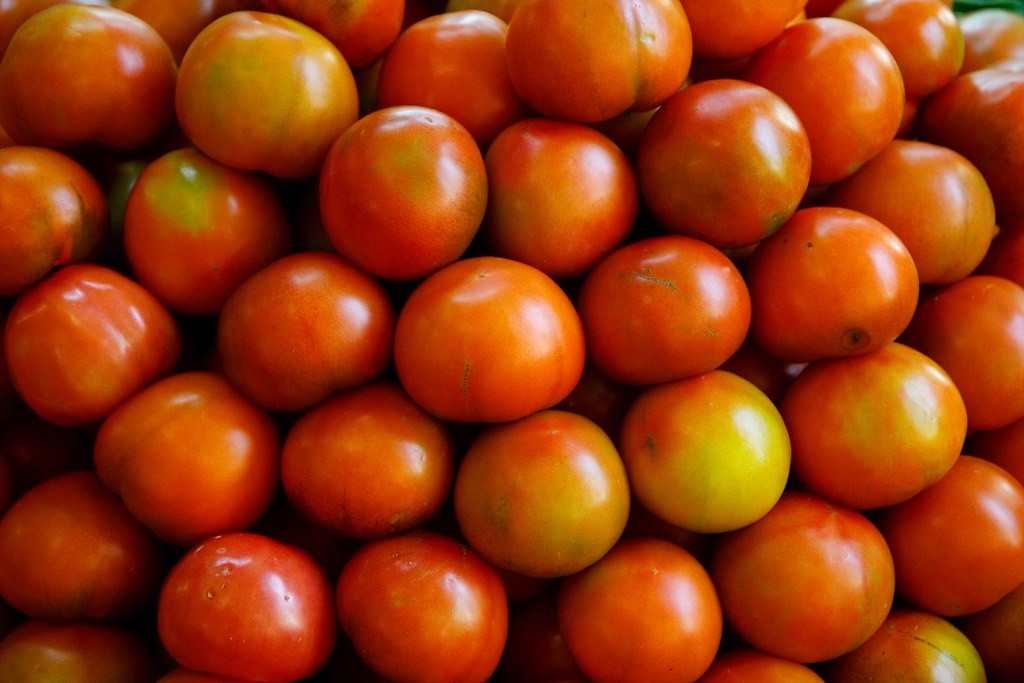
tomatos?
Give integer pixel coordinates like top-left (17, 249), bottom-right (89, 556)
top-left (0, 0), bottom-right (1024, 683)
